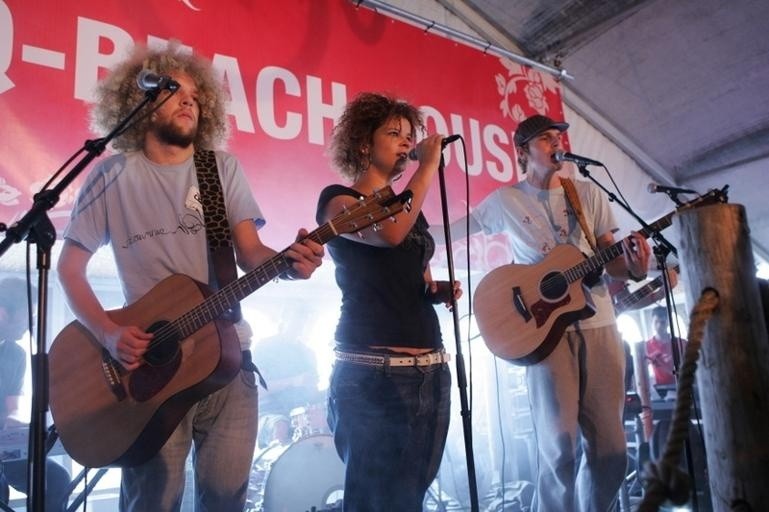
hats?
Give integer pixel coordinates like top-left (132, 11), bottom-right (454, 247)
top-left (515, 114), bottom-right (570, 148)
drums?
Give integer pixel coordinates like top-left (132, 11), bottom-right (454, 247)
top-left (290, 401), bottom-right (329, 439)
top-left (245, 433), bottom-right (347, 512)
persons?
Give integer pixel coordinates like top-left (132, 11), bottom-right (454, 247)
top-left (572, 263), bottom-right (682, 481)
top-left (312, 89), bottom-right (464, 512)
top-left (55, 38), bottom-right (326, 512)
top-left (424, 112), bottom-right (654, 511)
top-left (1, 272), bottom-right (73, 512)
top-left (246, 313), bottom-right (324, 457)
top-left (628, 302), bottom-right (689, 449)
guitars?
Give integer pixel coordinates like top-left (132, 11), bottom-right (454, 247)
top-left (48, 186), bottom-right (411, 466)
top-left (473, 185), bottom-right (730, 364)
top-left (610, 264), bottom-right (680, 320)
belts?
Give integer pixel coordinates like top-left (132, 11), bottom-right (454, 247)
top-left (334, 347), bottom-right (451, 368)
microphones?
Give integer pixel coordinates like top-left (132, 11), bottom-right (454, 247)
top-left (552, 145), bottom-right (600, 170)
top-left (644, 180), bottom-right (689, 199)
top-left (407, 134), bottom-right (463, 162)
top-left (133, 66), bottom-right (173, 92)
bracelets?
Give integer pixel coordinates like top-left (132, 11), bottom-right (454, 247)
top-left (625, 269), bottom-right (648, 284)
top-left (278, 272), bottom-right (293, 282)
